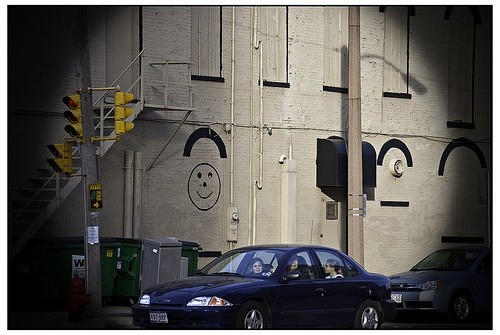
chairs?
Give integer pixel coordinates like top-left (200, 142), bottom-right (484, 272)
top-left (297, 256), bottom-right (313, 279)
top-left (263, 264), bottom-right (273, 273)
top-left (322, 264), bottom-right (348, 278)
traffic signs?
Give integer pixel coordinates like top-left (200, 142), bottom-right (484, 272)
top-left (89, 182), bottom-right (104, 211)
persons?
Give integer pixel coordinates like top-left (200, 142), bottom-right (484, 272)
top-left (324, 259), bottom-right (345, 279)
top-left (246, 258), bottom-right (270, 277)
top-left (286, 252), bottom-right (298, 272)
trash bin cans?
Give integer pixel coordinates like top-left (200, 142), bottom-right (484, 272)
top-left (114, 236), bottom-right (143, 307)
top-left (179, 240), bottom-right (203, 274)
top-left (26, 235), bottom-right (115, 311)
top-left (141, 235), bottom-right (182, 299)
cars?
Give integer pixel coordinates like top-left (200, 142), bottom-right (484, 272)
top-left (387, 244), bottom-right (493, 330)
top-left (131, 219), bottom-right (398, 330)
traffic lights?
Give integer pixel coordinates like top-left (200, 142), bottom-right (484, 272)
top-left (47, 144), bottom-right (72, 173)
top-left (62, 95), bottom-right (84, 139)
top-left (114, 91), bottom-right (135, 136)
